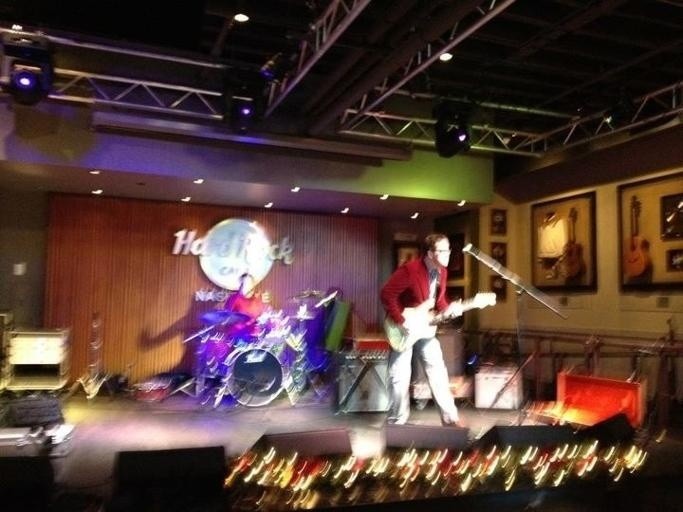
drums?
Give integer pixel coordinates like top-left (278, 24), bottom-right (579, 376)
top-left (224, 342), bottom-right (289, 406)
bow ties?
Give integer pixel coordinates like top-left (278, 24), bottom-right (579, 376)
top-left (429, 269), bottom-right (439, 282)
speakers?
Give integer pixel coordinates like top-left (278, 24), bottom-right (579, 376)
top-left (476, 424), bottom-right (575, 451)
top-left (385, 423), bottom-right (470, 451)
top-left (0, 398), bottom-right (63, 427)
top-left (115, 445), bottom-right (225, 512)
top-left (240, 429), bottom-right (354, 461)
top-left (575, 413), bottom-right (635, 448)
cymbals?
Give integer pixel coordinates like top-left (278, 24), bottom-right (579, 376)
top-left (203, 311), bottom-right (251, 323)
top-left (290, 291), bottom-right (321, 300)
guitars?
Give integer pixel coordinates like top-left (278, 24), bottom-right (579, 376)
top-left (621, 196), bottom-right (651, 278)
top-left (384, 293), bottom-right (496, 352)
top-left (559, 208), bottom-right (587, 283)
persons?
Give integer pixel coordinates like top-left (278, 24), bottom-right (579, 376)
top-left (378, 233), bottom-right (464, 428)
top-left (224, 273), bottom-right (271, 346)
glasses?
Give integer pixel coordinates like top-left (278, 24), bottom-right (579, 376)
top-left (433, 249), bottom-right (451, 255)
